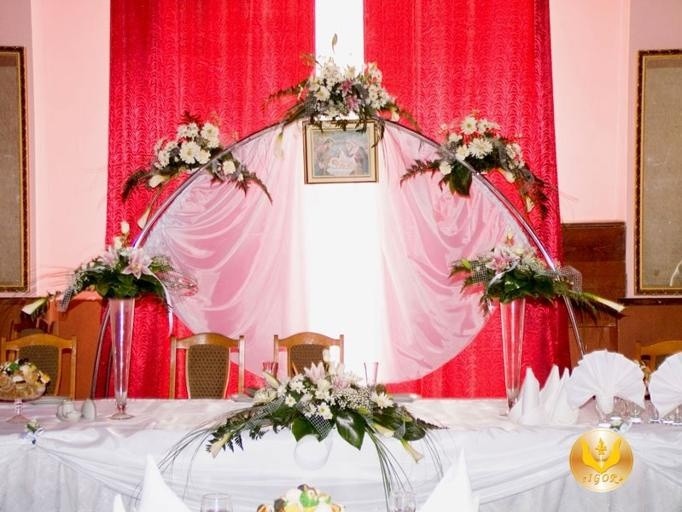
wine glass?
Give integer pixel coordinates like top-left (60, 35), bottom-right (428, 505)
top-left (0, 382), bottom-right (46, 425)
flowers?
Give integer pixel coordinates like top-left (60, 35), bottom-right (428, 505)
top-left (451, 232), bottom-right (626, 321)
top-left (400, 109), bottom-right (548, 222)
top-left (56, 220), bottom-right (197, 313)
top-left (134, 346), bottom-right (443, 511)
top-left (121, 112), bottom-right (272, 231)
top-left (259, 32), bottom-right (420, 150)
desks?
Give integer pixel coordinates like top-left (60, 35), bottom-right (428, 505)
top-left (0, 395), bottom-right (681, 512)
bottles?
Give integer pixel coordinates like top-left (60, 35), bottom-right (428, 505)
top-left (80, 397), bottom-right (98, 423)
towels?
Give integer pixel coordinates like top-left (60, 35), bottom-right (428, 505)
top-left (511, 364), bottom-right (579, 425)
top-left (565, 349), bottom-right (646, 415)
top-left (647, 352), bottom-right (681, 418)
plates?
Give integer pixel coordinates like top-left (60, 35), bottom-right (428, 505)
top-left (388, 393), bottom-right (422, 403)
top-left (229, 393), bottom-right (253, 403)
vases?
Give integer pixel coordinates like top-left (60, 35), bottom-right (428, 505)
top-left (499, 296), bottom-right (526, 416)
top-left (105, 298), bottom-right (139, 420)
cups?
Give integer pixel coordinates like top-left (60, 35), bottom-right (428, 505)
top-left (596, 396), bottom-right (682, 431)
top-left (67, 411), bottom-right (82, 422)
top-left (364, 360), bottom-right (379, 391)
top-left (262, 362), bottom-right (278, 382)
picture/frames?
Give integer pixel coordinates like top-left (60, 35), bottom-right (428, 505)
top-left (0, 45), bottom-right (31, 292)
top-left (302, 119), bottom-right (379, 184)
top-left (633, 47), bottom-right (682, 296)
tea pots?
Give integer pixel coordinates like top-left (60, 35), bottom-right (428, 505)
top-left (57, 398), bottom-right (75, 418)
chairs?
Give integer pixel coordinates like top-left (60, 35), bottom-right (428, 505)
top-left (634, 340), bottom-right (682, 394)
top-left (275, 332), bottom-right (344, 395)
top-left (1, 334), bottom-right (78, 402)
top-left (168, 332), bottom-right (245, 400)
top-left (8, 319), bottom-right (53, 363)
